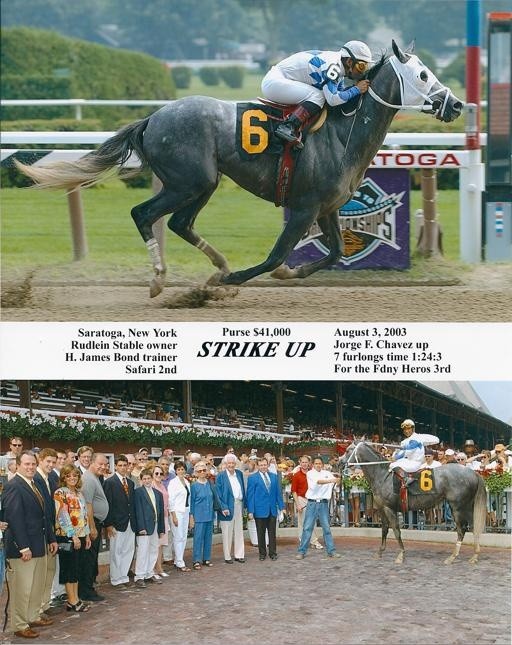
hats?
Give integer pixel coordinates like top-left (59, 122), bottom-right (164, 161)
top-left (444, 449), bottom-right (455, 456)
top-left (495, 444), bottom-right (505, 451)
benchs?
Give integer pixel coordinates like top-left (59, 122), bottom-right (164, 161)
top-left (1, 380), bottom-right (325, 437)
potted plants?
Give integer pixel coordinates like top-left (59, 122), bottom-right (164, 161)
top-left (484, 470), bottom-right (511, 496)
top-left (280, 476), bottom-right (291, 493)
top-left (341, 475), bottom-right (373, 496)
top-left (242, 509), bottom-right (269, 549)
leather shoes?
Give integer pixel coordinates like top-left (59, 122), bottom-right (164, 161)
top-left (14, 628), bottom-right (38, 637)
top-left (30, 615), bottom-right (53, 626)
top-left (88, 594), bottom-right (104, 601)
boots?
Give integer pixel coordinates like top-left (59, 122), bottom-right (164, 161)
top-left (395, 467), bottom-right (416, 487)
top-left (275, 101), bottom-right (322, 149)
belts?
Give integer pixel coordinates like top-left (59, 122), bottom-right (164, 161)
top-left (308, 499), bottom-right (328, 503)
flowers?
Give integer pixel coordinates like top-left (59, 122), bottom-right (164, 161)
top-left (1, 409), bottom-right (334, 458)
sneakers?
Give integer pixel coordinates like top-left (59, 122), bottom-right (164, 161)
top-left (135, 578), bottom-right (147, 588)
top-left (154, 572), bottom-right (170, 579)
top-left (329, 552), bottom-right (343, 558)
top-left (295, 553), bottom-right (304, 559)
top-left (270, 554), bottom-right (277, 560)
top-left (113, 582), bottom-right (136, 591)
top-left (259, 555), bottom-right (266, 561)
top-left (235, 557), bottom-right (245, 563)
top-left (145, 576), bottom-right (163, 584)
top-left (224, 560), bottom-right (234, 564)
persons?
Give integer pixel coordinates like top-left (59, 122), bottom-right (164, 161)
top-left (262, 40), bottom-right (374, 146)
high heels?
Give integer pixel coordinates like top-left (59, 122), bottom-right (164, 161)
top-left (174, 564), bottom-right (192, 572)
top-left (67, 599), bottom-right (88, 612)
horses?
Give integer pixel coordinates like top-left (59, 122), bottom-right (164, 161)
top-left (335, 433), bottom-right (487, 565)
top-left (12, 40), bottom-right (463, 299)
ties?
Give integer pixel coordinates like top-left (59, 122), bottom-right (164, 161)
top-left (122, 478), bottom-right (129, 495)
top-left (31, 481), bottom-right (45, 512)
top-left (263, 472), bottom-right (270, 489)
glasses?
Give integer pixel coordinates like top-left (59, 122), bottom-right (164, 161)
top-left (11, 443), bottom-right (22, 447)
top-left (154, 472), bottom-right (164, 476)
top-left (425, 455), bottom-right (432, 458)
top-left (481, 456), bottom-right (487, 459)
top-left (196, 469), bottom-right (206, 473)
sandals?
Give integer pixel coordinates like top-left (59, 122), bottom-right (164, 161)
top-left (203, 560), bottom-right (213, 567)
top-left (193, 562), bottom-right (202, 570)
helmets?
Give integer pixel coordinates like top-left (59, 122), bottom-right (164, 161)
top-left (341, 40), bottom-right (375, 63)
top-left (400, 419), bottom-right (416, 428)
